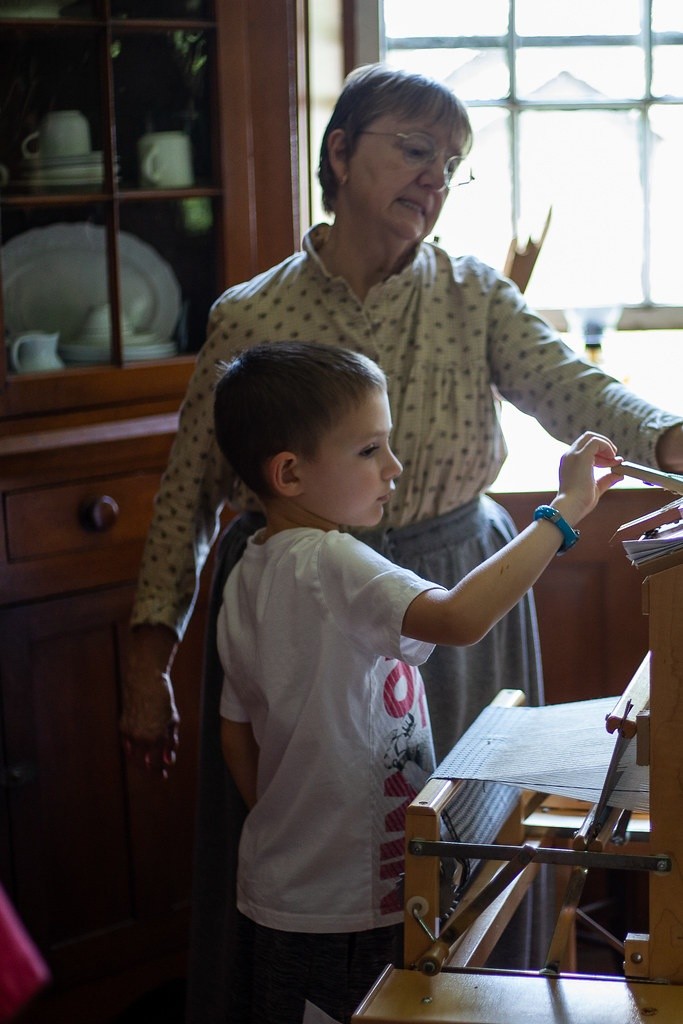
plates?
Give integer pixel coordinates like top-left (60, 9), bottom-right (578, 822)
top-left (0, 152), bottom-right (184, 363)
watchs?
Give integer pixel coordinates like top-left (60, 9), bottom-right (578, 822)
top-left (533, 505), bottom-right (581, 556)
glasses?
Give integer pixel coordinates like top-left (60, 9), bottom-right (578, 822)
top-left (354, 131), bottom-right (477, 187)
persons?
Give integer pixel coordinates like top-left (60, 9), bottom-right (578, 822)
top-left (214, 340), bottom-right (624, 1024)
top-left (118, 70), bottom-right (682, 1024)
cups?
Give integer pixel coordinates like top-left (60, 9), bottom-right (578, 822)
top-left (5, 328), bottom-right (66, 377)
top-left (137, 130), bottom-right (194, 190)
top-left (22, 112), bottom-right (91, 160)
